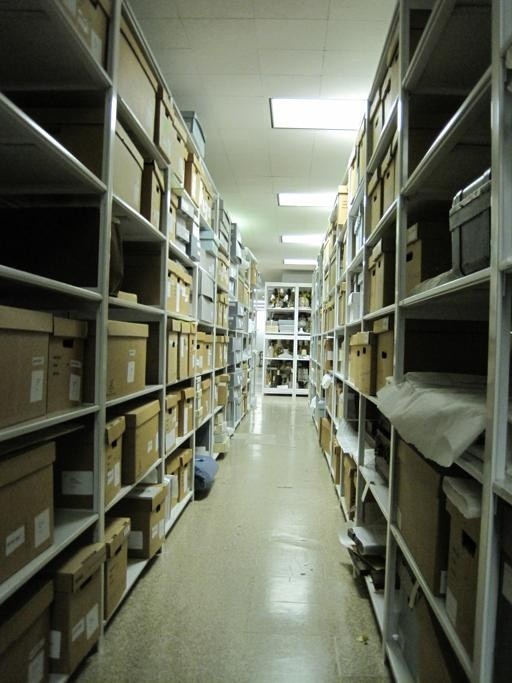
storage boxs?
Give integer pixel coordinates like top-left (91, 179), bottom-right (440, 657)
top-left (0, 0), bottom-right (258, 680)
top-left (305, 0), bottom-right (511, 679)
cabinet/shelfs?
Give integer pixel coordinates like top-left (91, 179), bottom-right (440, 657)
top-left (262, 281), bottom-right (316, 396)
